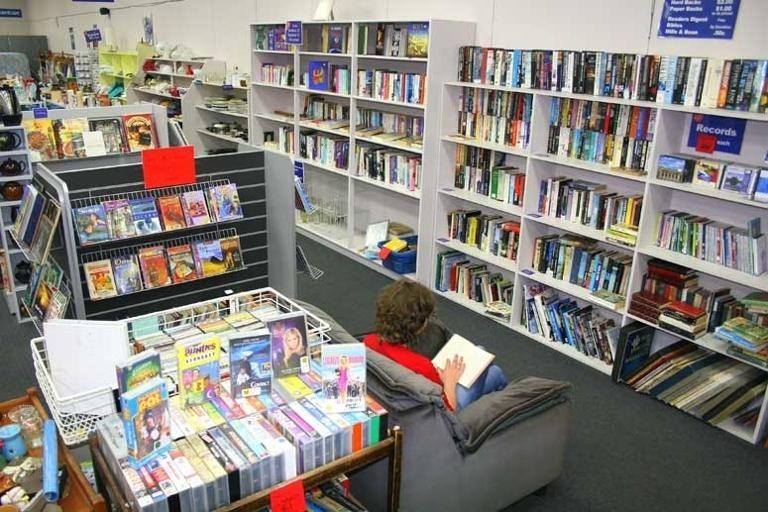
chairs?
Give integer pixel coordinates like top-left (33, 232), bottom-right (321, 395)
top-left (287, 294), bottom-right (573, 510)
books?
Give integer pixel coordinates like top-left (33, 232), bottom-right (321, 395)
top-left (255, 22), bottom-right (430, 191)
top-left (611, 320), bottom-right (768, 425)
top-left (26, 255), bottom-right (389, 512)
top-left (23, 113), bottom-right (243, 301)
top-left (435, 249), bottom-right (515, 323)
top-left (430, 333), bottom-right (496, 389)
top-left (364, 219), bottom-right (417, 261)
top-left (628, 258), bottom-right (768, 368)
top-left (458, 46), bottom-right (768, 312)
top-left (520, 282), bottom-right (621, 365)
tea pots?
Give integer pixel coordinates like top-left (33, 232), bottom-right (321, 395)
top-left (0, 131), bottom-right (32, 284)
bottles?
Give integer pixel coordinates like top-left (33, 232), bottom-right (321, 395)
top-left (0, 406), bottom-right (46, 462)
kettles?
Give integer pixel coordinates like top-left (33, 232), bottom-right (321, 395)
top-left (0, 84), bottom-right (22, 125)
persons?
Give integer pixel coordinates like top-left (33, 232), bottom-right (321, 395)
top-left (362, 275), bottom-right (506, 412)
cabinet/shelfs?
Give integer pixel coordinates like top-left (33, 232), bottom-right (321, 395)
top-left (432, 81), bottom-right (768, 444)
top-left (249, 19), bottom-right (477, 285)
top-left (42, 50), bottom-right (89, 88)
top-left (1, 386), bottom-right (109, 512)
top-left (126, 57), bottom-right (225, 145)
top-left (98, 49), bottom-right (153, 103)
top-left (194, 80), bottom-right (248, 150)
